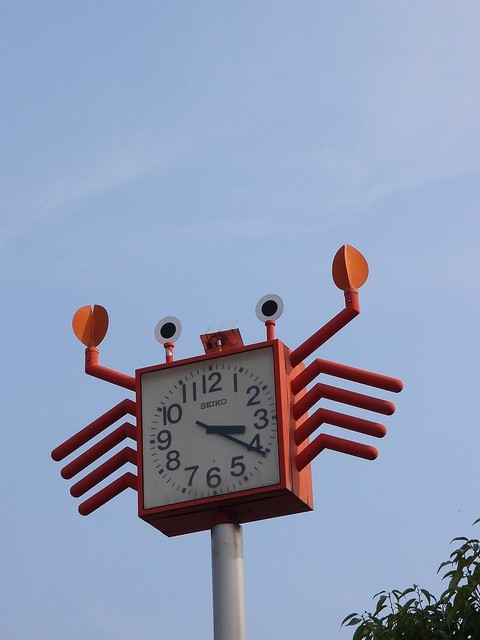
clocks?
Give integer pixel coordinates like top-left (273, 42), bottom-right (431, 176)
top-left (140, 345), bottom-right (281, 512)
